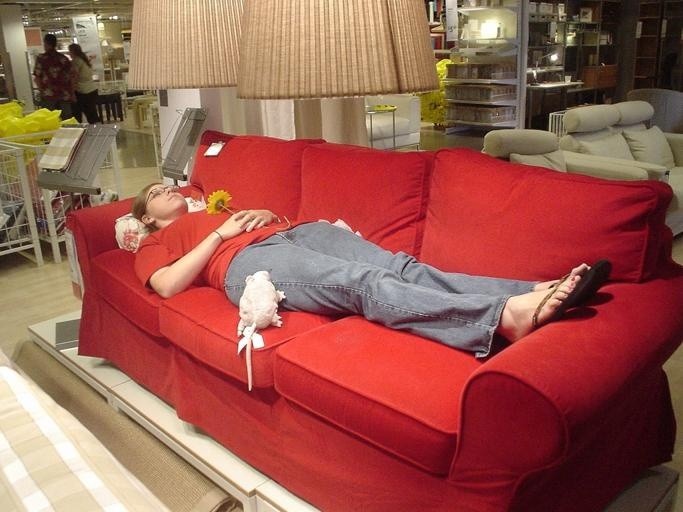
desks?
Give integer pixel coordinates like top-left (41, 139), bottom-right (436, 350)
top-left (96, 88), bottom-right (125, 124)
top-left (527, 81), bottom-right (584, 129)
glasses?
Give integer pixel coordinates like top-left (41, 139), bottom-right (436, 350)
top-left (146, 184), bottom-right (180, 205)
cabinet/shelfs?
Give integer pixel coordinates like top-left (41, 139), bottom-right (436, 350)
top-left (526, 3), bottom-right (565, 78)
top-left (564, 20), bottom-right (601, 107)
top-left (657, 1), bottom-right (683, 91)
top-left (443, 4), bottom-right (522, 130)
top-left (631, 1), bottom-right (663, 88)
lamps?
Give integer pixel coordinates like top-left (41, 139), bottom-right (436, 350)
top-left (234, 1), bottom-right (442, 103)
top-left (530, 50), bottom-right (559, 87)
top-left (126, 1), bottom-right (242, 90)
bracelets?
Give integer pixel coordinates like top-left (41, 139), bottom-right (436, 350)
top-left (213, 231), bottom-right (224, 242)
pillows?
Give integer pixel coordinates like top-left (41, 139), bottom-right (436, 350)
top-left (507, 149), bottom-right (569, 176)
top-left (578, 133), bottom-right (635, 165)
top-left (622, 125), bottom-right (676, 172)
top-left (109, 196), bottom-right (205, 253)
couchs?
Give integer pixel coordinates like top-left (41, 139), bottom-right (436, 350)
top-left (364, 94), bottom-right (422, 153)
top-left (626, 87), bottom-right (683, 133)
top-left (560, 99), bottom-right (683, 238)
top-left (481, 126), bottom-right (649, 188)
top-left (65, 129), bottom-right (683, 509)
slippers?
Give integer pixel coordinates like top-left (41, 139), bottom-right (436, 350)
top-left (528, 269), bottom-right (596, 327)
top-left (549, 259), bottom-right (612, 300)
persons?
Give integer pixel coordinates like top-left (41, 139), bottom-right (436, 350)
top-left (68, 43), bottom-right (100, 123)
top-left (131, 182), bottom-right (612, 360)
top-left (32, 34), bottom-right (72, 121)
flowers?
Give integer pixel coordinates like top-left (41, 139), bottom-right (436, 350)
top-left (204, 188), bottom-right (236, 219)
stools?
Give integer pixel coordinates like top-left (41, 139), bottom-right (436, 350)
top-left (130, 95), bottom-right (155, 129)
top-left (363, 105), bottom-right (397, 152)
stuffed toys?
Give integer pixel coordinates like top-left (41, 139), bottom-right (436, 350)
top-left (237, 270), bottom-right (285, 391)
